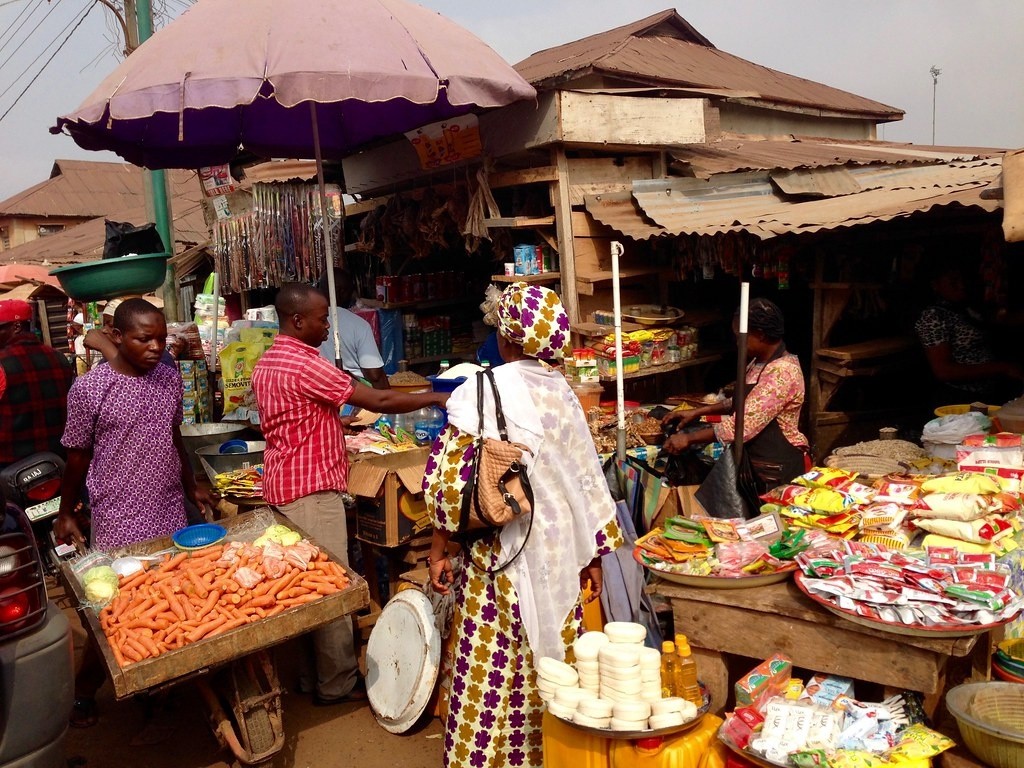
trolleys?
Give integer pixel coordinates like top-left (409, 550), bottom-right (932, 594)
top-left (63, 497), bottom-right (371, 768)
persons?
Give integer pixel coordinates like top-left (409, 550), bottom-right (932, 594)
top-left (914, 257), bottom-right (1024, 417)
top-left (310, 266), bottom-right (391, 392)
top-left (250, 282), bottom-right (451, 702)
top-left (1, 299), bottom-right (77, 531)
top-left (661, 298), bottom-right (816, 497)
top-left (83, 299), bottom-right (177, 370)
top-left (423, 281), bottom-right (624, 768)
top-left (53, 298), bottom-right (217, 731)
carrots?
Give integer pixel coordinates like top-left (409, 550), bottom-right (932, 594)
top-left (98, 542), bottom-right (351, 669)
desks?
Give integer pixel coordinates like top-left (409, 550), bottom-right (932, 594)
top-left (642, 577), bottom-right (985, 728)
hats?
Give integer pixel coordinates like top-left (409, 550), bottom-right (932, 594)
top-left (0, 299), bottom-right (33, 325)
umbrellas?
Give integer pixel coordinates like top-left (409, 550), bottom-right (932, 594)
top-left (52, 0), bottom-right (539, 372)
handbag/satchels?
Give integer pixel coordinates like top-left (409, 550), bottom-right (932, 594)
top-left (466, 370), bottom-right (532, 530)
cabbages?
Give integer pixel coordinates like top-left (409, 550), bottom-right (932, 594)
top-left (82, 565), bottom-right (121, 602)
top-left (252, 525), bottom-right (302, 547)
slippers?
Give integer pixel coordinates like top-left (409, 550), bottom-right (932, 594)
top-left (313, 686), bottom-right (369, 706)
top-left (136, 692), bottom-right (170, 714)
top-left (68, 694), bottom-right (99, 728)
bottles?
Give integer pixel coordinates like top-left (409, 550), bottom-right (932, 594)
top-left (480, 360), bottom-right (491, 370)
top-left (659, 633), bottom-right (705, 710)
top-left (437, 361), bottom-right (450, 378)
top-left (375, 403), bottom-right (445, 447)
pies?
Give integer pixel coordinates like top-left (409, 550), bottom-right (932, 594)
top-left (537, 621), bottom-right (698, 732)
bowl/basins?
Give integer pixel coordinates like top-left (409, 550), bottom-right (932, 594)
top-left (181, 423), bottom-right (247, 481)
top-left (391, 383), bottom-right (429, 395)
top-left (195, 441), bottom-right (266, 488)
top-left (47, 252), bottom-right (173, 303)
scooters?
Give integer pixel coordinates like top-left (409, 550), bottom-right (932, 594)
top-left (2, 450), bottom-right (92, 589)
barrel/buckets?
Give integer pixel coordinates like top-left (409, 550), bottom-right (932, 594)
top-left (425, 375), bottom-right (466, 425)
top-left (374, 271), bottom-right (476, 304)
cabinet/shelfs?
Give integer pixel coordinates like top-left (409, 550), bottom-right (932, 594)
top-left (344, 240), bottom-right (483, 368)
top-left (570, 213), bottom-right (920, 469)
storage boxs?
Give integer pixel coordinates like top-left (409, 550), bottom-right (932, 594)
top-left (346, 448), bottom-right (434, 548)
